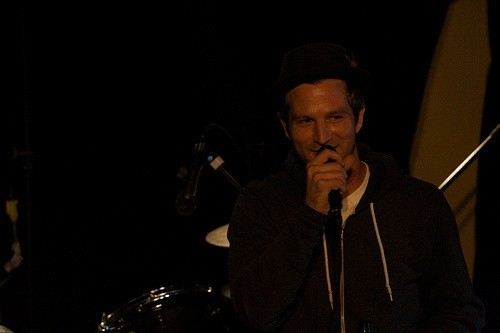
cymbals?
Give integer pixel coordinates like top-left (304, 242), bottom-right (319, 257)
top-left (206, 223), bottom-right (231, 248)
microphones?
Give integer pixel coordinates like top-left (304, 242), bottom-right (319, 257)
top-left (175, 136), bottom-right (206, 216)
top-left (318, 144), bottom-right (343, 223)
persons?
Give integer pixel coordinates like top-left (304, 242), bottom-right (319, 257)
top-left (227, 49), bottom-right (486, 333)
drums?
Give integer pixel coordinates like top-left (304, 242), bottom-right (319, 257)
top-left (98, 286), bottom-right (231, 333)
